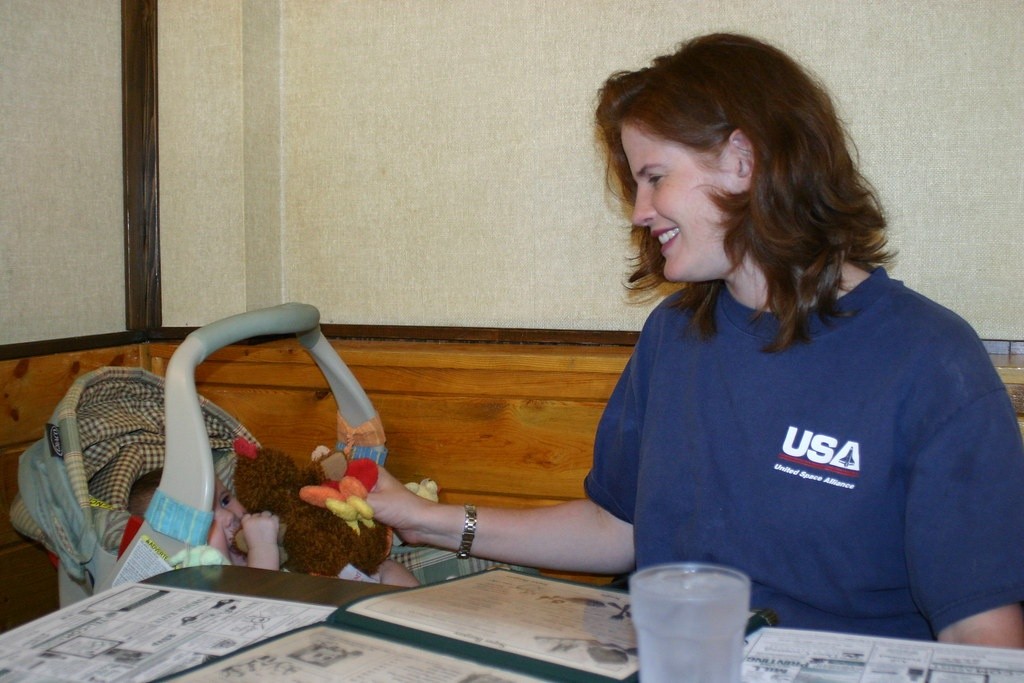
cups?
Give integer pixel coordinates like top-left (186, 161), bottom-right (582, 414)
top-left (629, 563), bottom-right (751, 683)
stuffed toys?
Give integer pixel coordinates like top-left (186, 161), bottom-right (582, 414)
top-left (229, 436), bottom-right (396, 578)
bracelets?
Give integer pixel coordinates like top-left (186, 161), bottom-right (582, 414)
top-left (456, 504), bottom-right (477, 559)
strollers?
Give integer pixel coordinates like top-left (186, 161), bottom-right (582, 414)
top-left (7, 303), bottom-right (541, 619)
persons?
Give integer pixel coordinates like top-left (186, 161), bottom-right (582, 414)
top-left (362, 33), bottom-right (1024, 650)
top-left (127, 466), bottom-right (421, 588)
